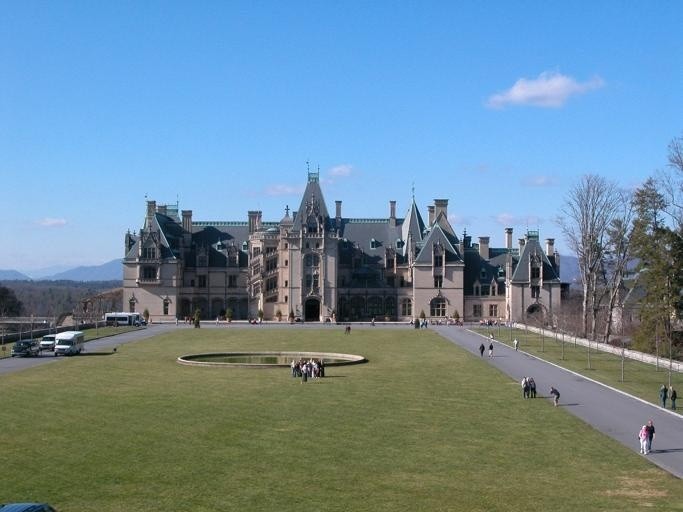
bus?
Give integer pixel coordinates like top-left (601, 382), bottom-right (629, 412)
top-left (105, 312), bottom-right (148, 327)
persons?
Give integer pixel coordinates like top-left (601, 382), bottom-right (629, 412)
top-left (521, 376), bottom-right (536, 399)
top-left (659, 384), bottom-right (676, 410)
top-left (344, 324), bottom-right (350, 335)
top-left (290, 359), bottom-right (324, 384)
top-left (638, 420), bottom-right (655, 455)
top-left (512, 338), bottom-right (519, 351)
top-left (215, 315), bottom-right (219, 327)
top-left (489, 343), bottom-right (493, 358)
top-left (479, 344), bottom-right (484, 357)
top-left (550, 386), bottom-right (560, 407)
top-left (409, 316), bottom-right (464, 329)
top-left (479, 319), bottom-right (500, 328)
top-left (490, 332), bottom-right (495, 343)
top-left (184, 315), bottom-right (194, 324)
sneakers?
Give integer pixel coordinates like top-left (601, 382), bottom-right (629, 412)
top-left (640, 451), bottom-right (650, 455)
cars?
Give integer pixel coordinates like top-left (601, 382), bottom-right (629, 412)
top-left (11, 331), bottom-right (84, 358)
